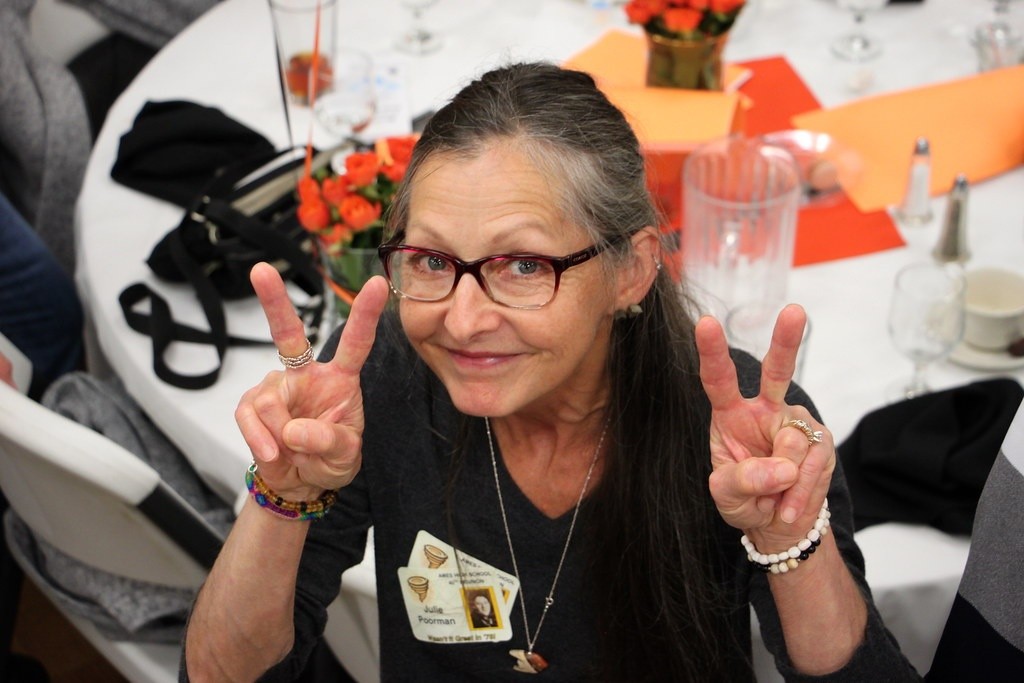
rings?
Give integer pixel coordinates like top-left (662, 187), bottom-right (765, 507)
top-left (786, 419), bottom-right (823, 446)
top-left (277, 338), bottom-right (314, 368)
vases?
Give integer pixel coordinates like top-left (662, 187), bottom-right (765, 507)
top-left (647, 32), bottom-right (729, 90)
top-left (320, 238), bottom-right (393, 329)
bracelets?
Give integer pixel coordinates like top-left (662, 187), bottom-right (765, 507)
top-left (245, 462), bottom-right (336, 520)
top-left (740, 496), bottom-right (830, 575)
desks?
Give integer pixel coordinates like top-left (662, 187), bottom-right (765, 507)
top-left (75, 1), bottom-right (1024, 682)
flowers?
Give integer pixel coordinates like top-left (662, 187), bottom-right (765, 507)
top-left (299, 131), bottom-right (421, 291)
top-left (622, 1), bottom-right (744, 90)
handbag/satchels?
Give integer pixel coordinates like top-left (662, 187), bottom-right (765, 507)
top-left (146, 144), bottom-right (335, 302)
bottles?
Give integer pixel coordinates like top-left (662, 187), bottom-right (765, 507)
top-left (931, 176), bottom-right (974, 268)
top-left (899, 138), bottom-right (934, 227)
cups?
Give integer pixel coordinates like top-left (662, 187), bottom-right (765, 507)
top-left (309, 46), bottom-right (374, 148)
top-left (679, 137), bottom-right (799, 346)
top-left (725, 297), bottom-right (812, 392)
top-left (269, 0), bottom-right (361, 158)
top-left (966, 266), bottom-right (1024, 350)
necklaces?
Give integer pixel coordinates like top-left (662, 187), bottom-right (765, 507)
top-left (485, 416), bottom-right (610, 674)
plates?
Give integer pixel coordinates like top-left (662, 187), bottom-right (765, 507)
top-left (950, 340), bottom-right (1024, 372)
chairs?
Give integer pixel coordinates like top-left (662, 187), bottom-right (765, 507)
top-left (28, 0), bottom-right (161, 145)
top-left (0, 380), bottom-right (225, 587)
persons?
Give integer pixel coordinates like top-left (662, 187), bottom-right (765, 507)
top-left (0, 189), bottom-right (85, 683)
top-left (471, 595), bottom-right (497, 628)
top-left (179, 63), bottom-right (881, 683)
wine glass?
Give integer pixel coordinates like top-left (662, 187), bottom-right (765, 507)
top-left (889, 264), bottom-right (961, 399)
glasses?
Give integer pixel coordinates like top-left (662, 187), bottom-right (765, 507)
top-left (379, 223), bottom-right (646, 310)
